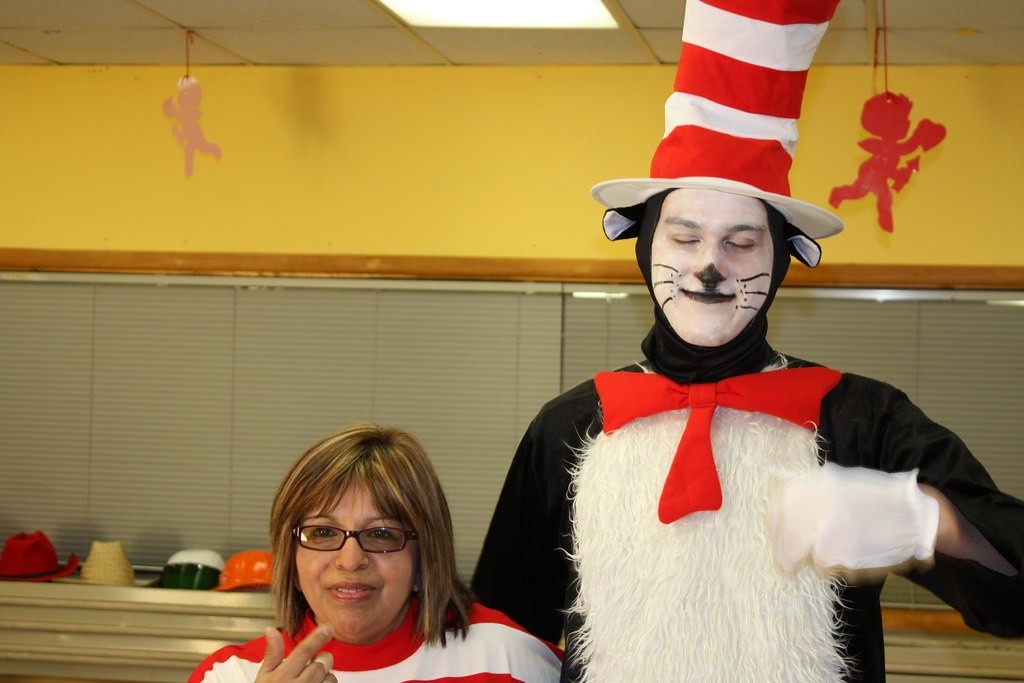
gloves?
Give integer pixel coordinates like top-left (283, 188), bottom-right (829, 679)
top-left (767, 464), bottom-right (939, 571)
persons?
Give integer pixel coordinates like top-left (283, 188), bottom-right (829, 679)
top-left (186, 424), bottom-right (563, 683)
top-left (470, 186), bottom-right (1024, 683)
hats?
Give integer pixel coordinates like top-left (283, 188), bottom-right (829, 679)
top-left (0, 530), bottom-right (80, 582)
top-left (50, 539), bottom-right (161, 587)
top-left (589, 0), bottom-right (840, 240)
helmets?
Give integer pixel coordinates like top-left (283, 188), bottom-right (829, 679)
top-left (149, 548), bottom-right (225, 590)
top-left (211, 550), bottom-right (276, 592)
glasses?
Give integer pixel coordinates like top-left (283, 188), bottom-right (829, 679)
top-left (290, 526), bottom-right (416, 554)
